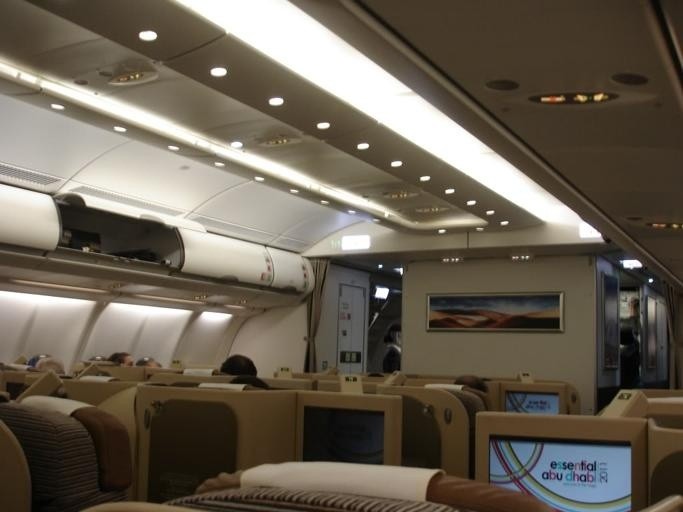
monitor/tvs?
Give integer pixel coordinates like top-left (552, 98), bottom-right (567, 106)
top-left (295, 390), bottom-right (404, 465)
top-left (474, 411), bottom-right (647, 512)
top-left (500, 382), bottom-right (581, 414)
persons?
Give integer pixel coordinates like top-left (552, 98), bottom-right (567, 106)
top-left (380, 334), bottom-right (401, 373)
top-left (619, 295), bottom-right (643, 389)
top-left (106, 351), bottom-right (134, 366)
top-left (135, 355), bottom-right (162, 368)
top-left (219, 353), bottom-right (257, 376)
